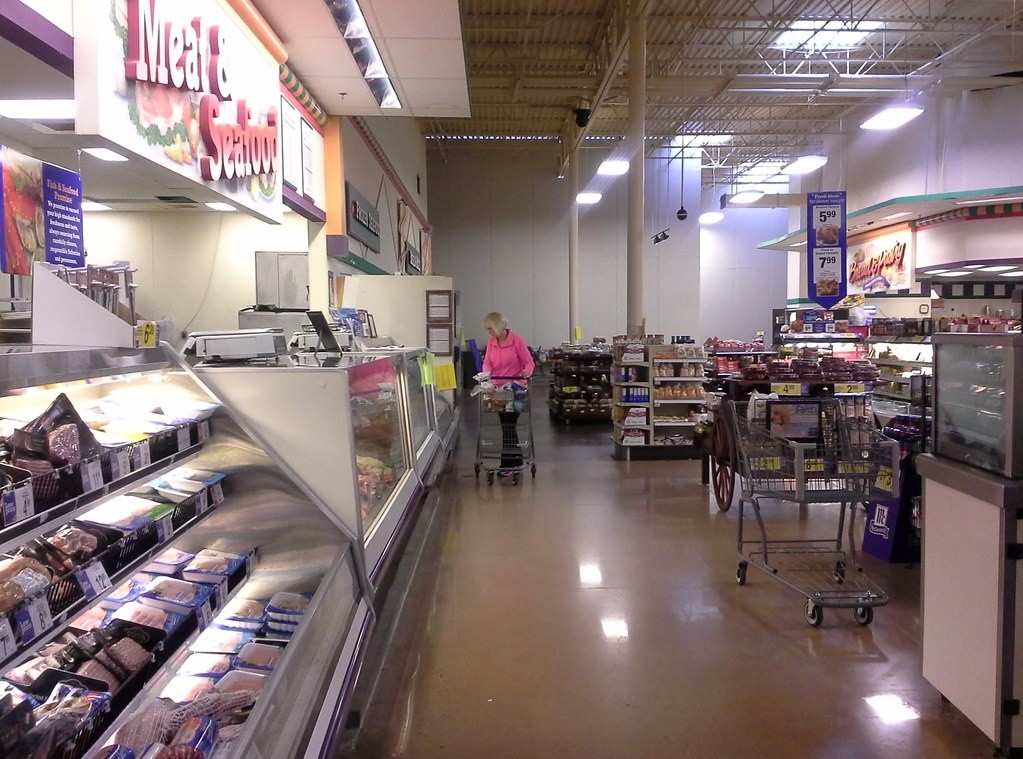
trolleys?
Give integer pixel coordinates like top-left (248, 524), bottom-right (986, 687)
top-left (474, 378), bottom-right (537, 485)
top-left (729, 397), bottom-right (901, 628)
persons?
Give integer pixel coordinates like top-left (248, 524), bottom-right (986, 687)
top-left (483, 312), bottom-right (535, 478)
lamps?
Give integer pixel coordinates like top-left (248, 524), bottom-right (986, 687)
top-left (651, 228), bottom-right (670, 245)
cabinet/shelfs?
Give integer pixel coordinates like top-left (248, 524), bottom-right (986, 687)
top-left (720, 376), bottom-right (876, 422)
top-left (868, 334), bottom-right (932, 454)
top-left (916, 453), bottom-right (1023, 759)
top-left (773, 307), bottom-right (868, 364)
top-left (703, 349), bottom-right (779, 438)
top-left (0, 342), bottom-right (377, 758)
top-left (609, 342), bottom-right (710, 460)
top-left (545, 343), bottom-right (615, 427)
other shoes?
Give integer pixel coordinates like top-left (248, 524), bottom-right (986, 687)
top-left (497, 463), bottom-right (524, 477)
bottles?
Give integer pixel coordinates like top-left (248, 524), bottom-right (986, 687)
top-left (612, 365), bottom-right (649, 403)
